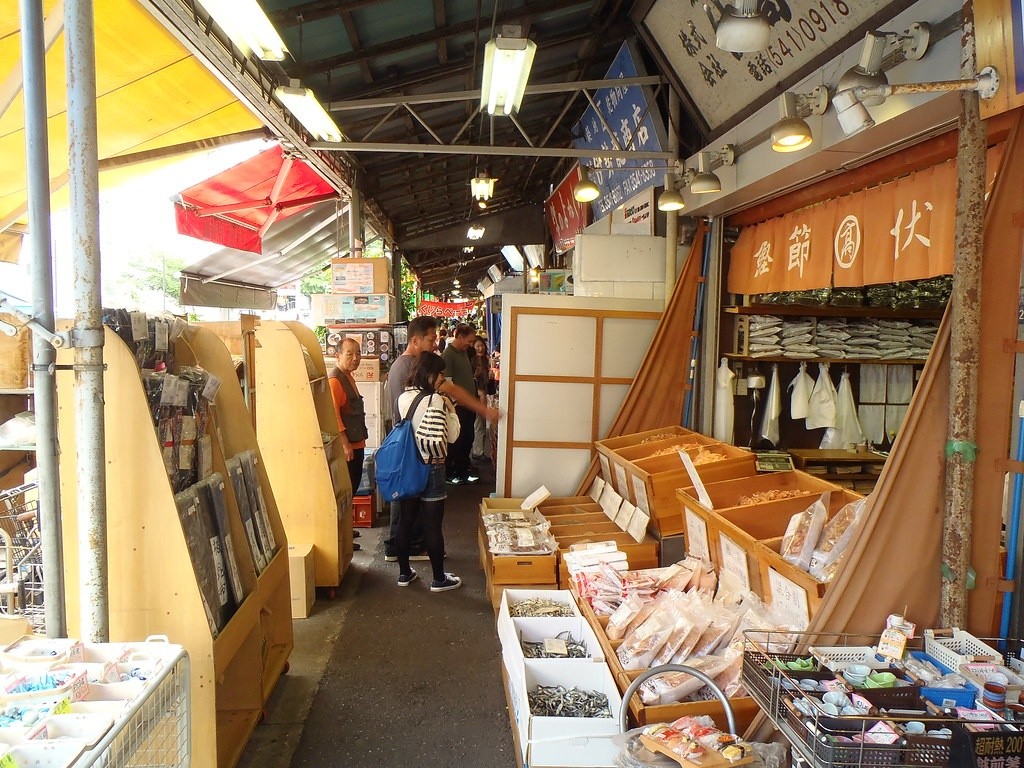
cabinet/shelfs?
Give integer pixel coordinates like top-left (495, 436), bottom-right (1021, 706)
top-left (725, 305), bottom-right (947, 488)
top-left (0, 309), bottom-right (354, 768)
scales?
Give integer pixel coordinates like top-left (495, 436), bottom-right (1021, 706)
top-left (752, 449), bottom-right (795, 472)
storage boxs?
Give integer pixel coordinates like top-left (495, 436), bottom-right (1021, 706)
top-left (321, 257), bottom-right (407, 529)
top-left (477, 422), bottom-right (1024, 768)
top-left (286, 542), bottom-right (316, 619)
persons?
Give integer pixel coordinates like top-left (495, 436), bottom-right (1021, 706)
top-left (328, 338), bottom-right (368, 551)
top-left (384, 315), bottom-right (499, 560)
top-left (434, 314), bottom-right (474, 355)
top-left (395, 351), bottom-right (462, 593)
top-left (474, 336), bottom-right (492, 461)
top-left (441, 324), bottom-right (480, 484)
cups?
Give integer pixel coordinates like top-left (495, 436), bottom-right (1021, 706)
top-left (890, 614), bottom-right (904, 626)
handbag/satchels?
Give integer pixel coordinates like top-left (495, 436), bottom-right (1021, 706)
top-left (485, 380), bottom-right (496, 395)
top-left (415, 393), bottom-right (448, 458)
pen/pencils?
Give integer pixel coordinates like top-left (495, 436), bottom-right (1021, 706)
top-left (904, 605), bottom-right (908, 617)
top-left (879, 452), bottom-right (888, 456)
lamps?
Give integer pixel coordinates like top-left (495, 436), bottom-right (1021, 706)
top-left (574, 158), bottom-right (684, 203)
top-left (658, 168), bottom-right (695, 211)
top-left (470, 173), bottom-right (499, 201)
top-left (832, 66), bottom-right (999, 137)
top-left (478, 201), bottom-right (488, 208)
top-left (690, 142), bottom-right (735, 194)
top-left (503, 263), bottom-right (566, 284)
top-left (835, 20), bottom-right (930, 105)
top-left (462, 223), bottom-right (485, 254)
top-left (197, 0), bottom-right (297, 63)
top-left (274, 77), bottom-right (352, 144)
top-left (772, 86), bottom-right (828, 153)
top-left (479, 23), bottom-right (537, 117)
top-left (715, 0), bottom-right (771, 52)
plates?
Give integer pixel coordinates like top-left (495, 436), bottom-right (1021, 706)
top-left (327, 346), bottom-right (336, 355)
top-left (380, 344), bottom-right (389, 352)
top-left (381, 353), bottom-right (388, 360)
top-left (367, 340), bottom-right (375, 347)
top-left (366, 332), bottom-right (375, 339)
top-left (367, 347), bottom-right (375, 352)
top-left (327, 333), bottom-right (342, 346)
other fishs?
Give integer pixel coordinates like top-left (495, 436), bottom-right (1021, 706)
top-left (527, 684), bottom-right (612, 719)
top-left (518, 630), bottom-right (591, 659)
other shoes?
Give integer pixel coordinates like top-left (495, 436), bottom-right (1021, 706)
top-left (459, 470), bottom-right (479, 483)
top-left (471, 452), bottom-right (491, 463)
top-left (352, 543), bottom-right (361, 551)
top-left (409, 549), bottom-right (447, 560)
top-left (384, 553), bottom-right (396, 561)
top-left (445, 474), bottom-right (463, 484)
top-left (352, 529), bottom-right (360, 538)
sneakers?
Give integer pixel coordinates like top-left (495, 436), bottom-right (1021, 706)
top-left (397, 571), bottom-right (417, 586)
top-left (430, 575), bottom-right (461, 592)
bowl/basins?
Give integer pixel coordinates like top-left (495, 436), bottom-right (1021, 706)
top-left (801, 691), bottom-right (866, 719)
top-left (901, 721), bottom-right (952, 762)
top-left (841, 664), bottom-right (896, 689)
top-left (982, 680), bottom-right (1024, 720)
top-left (767, 677), bottom-right (819, 694)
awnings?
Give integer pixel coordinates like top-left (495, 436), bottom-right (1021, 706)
top-left (173, 144), bottom-right (340, 254)
top-left (0, 0), bottom-right (271, 261)
top-left (179, 198), bottom-right (380, 309)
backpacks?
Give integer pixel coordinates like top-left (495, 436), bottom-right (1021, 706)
top-left (373, 390), bottom-right (435, 503)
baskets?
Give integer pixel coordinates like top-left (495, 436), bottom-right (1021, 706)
top-left (741, 626), bottom-right (1024, 768)
